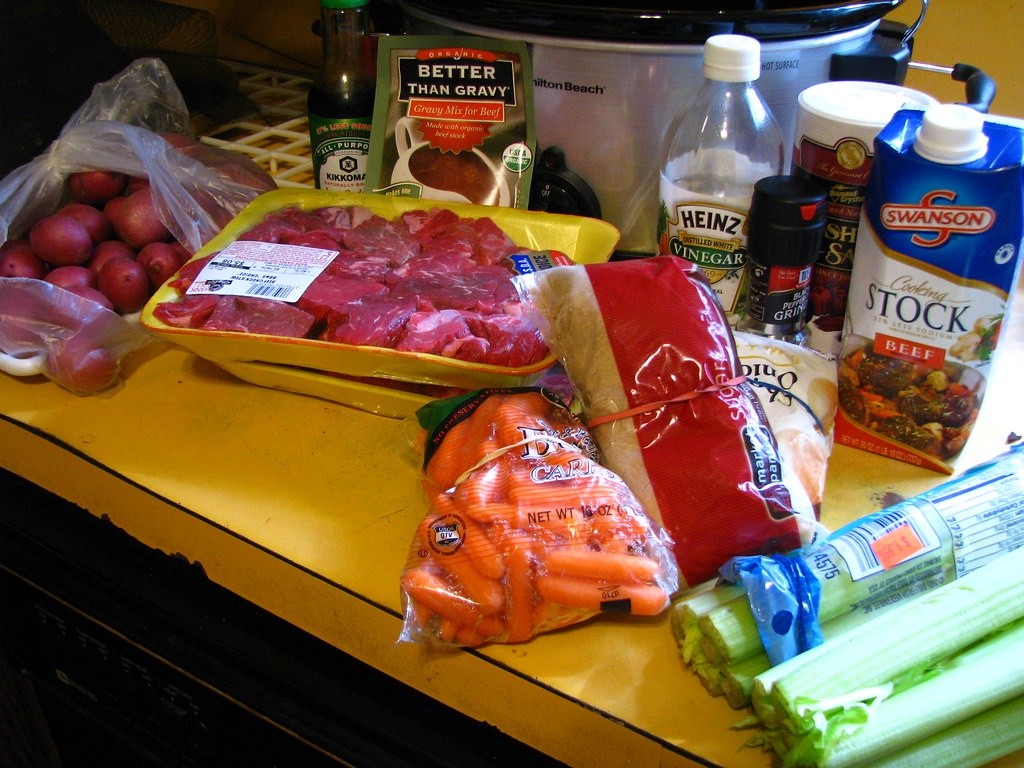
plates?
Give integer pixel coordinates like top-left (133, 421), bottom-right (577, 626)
top-left (141, 187), bottom-right (621, 389)
top-left (220, 362), bottom-right (581, 424)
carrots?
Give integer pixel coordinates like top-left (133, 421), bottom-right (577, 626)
top-left (398, 392), bottom-right (669, 647)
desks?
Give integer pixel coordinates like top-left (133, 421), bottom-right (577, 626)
top-left (1, 101), bottom-right (1024, 768)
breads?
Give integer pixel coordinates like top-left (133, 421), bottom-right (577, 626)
top-left (533, 265), bottom-right (818, 597)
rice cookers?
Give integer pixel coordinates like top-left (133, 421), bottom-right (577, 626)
top-left (312, 0), bottom-right (996, 261)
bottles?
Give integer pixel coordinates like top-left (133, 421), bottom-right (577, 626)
top-left (656, 35), bottom-right (784, 329)
top-left (309, 0), bottom-right (377, 192)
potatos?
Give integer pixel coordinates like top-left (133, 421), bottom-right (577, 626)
top-left (0, 129), bottom-right (281, 392)
